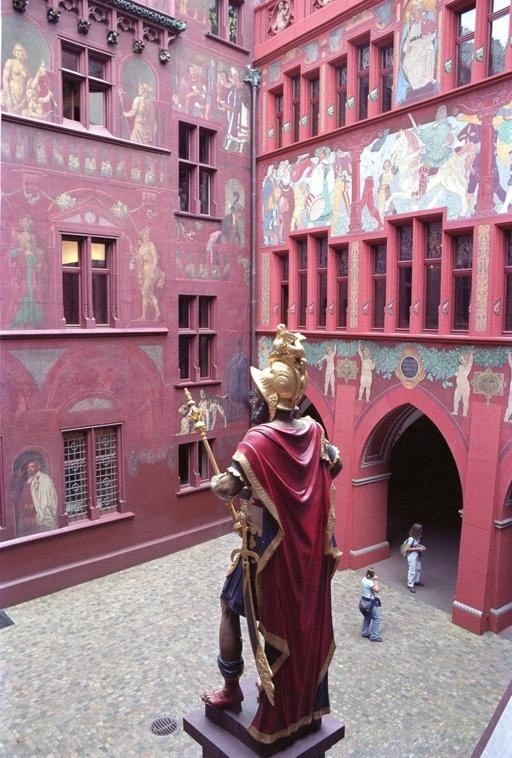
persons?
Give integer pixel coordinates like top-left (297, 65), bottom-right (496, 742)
top-left (403, 523), bottom-right (427, 592)
top-left (122, 83), bottom-right (157, 145)
top-left (360, 567), bottom-right (383, 643)
top-left (357, 343), bottom-right (376, 401)
top-left (25, 62), bottom-right (56, 116)
top-left (17, 460), bottom-right (58, 537)
top-left (449, 351), bottom-right (475, 417)
top-left (129, 228), bottom-right (164, 322)
top-left (175, 322), bottom-right (344, 746)
top-left (173, 55), bottom-right (251, 154)
top-left (2, 42), bottom-right (29, 113)
top-left (401, 0), bottom-right (437, 88)
top-left (224, 190), bottom-right (244, 248)
top-left (261, 104), bottom-right (512, 245)
top-left (316, 344), bottom-right (336, 398)
top-left (8, 215), bottom-right (50, 329)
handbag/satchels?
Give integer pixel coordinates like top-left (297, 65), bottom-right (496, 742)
top-left (359, 599), bottom-right (371, 615)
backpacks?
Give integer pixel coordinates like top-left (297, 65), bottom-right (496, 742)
top-left (400, 536), bottom-right (415, 558)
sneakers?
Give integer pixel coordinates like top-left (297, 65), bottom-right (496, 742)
top-left (370, 637), bottom-right (383, 642)
top-left (414, 582), bottom-right (424, 587)
top-left (408, 586), bottom-right (415, 592)
top-left (362, 633), bottom-right (369, 638)
